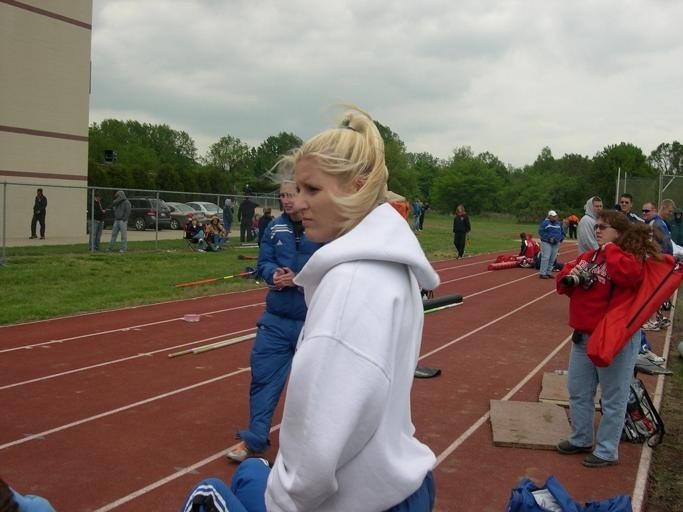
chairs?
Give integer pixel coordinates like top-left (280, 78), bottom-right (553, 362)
top-left (182, 222), bottom-right (231, 254)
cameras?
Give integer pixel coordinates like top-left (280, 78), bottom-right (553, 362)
top-left (559, 265), bottom-right (597, 292)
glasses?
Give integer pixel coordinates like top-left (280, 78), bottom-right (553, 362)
top-left (594, 223), bottom-right (613, 230)
top-left (642, 209), bottom-right (653, 213)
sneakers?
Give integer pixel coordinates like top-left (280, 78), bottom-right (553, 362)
top-left (582, 454), bottom-right (620, 468)
top-left (641, 350), bottom-right (665, 366)
top-left (641, 317), bottom-right (671, 332)
top-left (226, 441), bottom-right (251, 463)
top-left (557, 441), bottom-right (594, 455)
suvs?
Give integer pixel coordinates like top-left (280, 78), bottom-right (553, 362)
top-left (103, 198), bottom-right (171, 231)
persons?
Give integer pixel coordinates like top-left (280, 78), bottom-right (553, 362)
top-left (185, 193), bottom-right (276, 253)
top-left (419, 202), bottom-right (429, 230)
top-left (519, 193), bottom-right (683, 354)
top-left (0, 478), bottom-right (58, 512)
top-left (411, 195), bottom-right (422, 231)
top-left (106, 191), bottom-right (131, 253)
top-left (179, 101), bottom-right (441, 512)
top-left (29, 189), bottom-right (47, 239)
top-left (226, 179), bottom-right (324, 463)
top-left (88, 192), bottom-right (110, 250)
top-left (453, 204), bottom-right (472, 260)
top-left (556, 208), bottom-right (665, 467)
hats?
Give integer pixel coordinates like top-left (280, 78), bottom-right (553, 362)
top-left (549, 211), bottom-right (557, 217)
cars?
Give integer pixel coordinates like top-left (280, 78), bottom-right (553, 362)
top-left (182, 202), bottom-right (224, 226)
top-left (165, 201), bottom-right (206, 229)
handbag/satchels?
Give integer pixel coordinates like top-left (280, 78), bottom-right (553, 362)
top-left (505, 475), bottom-right (579, 512)
